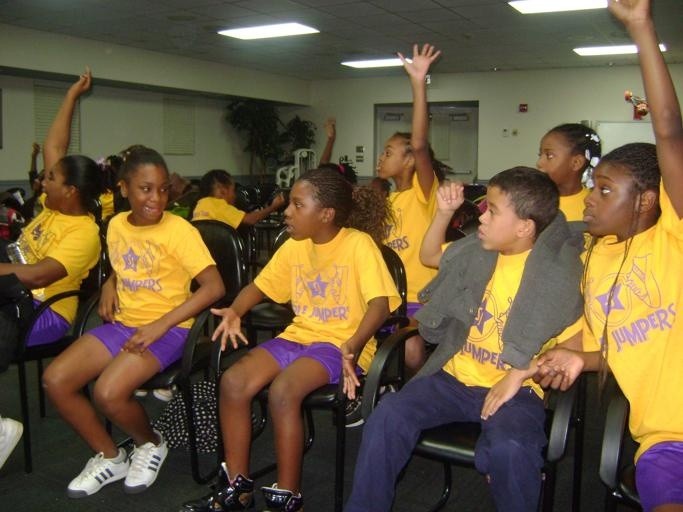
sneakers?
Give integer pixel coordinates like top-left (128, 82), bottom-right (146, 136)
top-left (181, 460), bottom-right (257, 512)
top-left (123, 426), bottom-right (169, 494)
top-left (67, 443), bottom-right (130, 499)
top-left (331, 382), bottom-right (398, 429)
top-left (261, 482), bottom-right (305, 512)
top-left (0, 415), bottom-right (24, 470)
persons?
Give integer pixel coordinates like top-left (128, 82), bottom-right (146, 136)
top-left (530, 0), bottom-right (682, 511)
top-left (176, 166), bottom-right (405, 511)
top-left (191, 168), bottom-right (284, 232)
top-left (340, 164), bottom-right (586, 511)
top-left (0, 62), bottom-right (118, 470)
top-left (537, 122), bottom-right (603, 221)
top-left (31, 142), bottom-right (227, 502)
top-left (97, 154), bottom-right (123, 220)
top-left (332, 43), bottom-right (443, 436)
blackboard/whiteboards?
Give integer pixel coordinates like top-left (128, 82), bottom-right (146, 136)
top-left (590, 120), bottom-right (657, 158)
